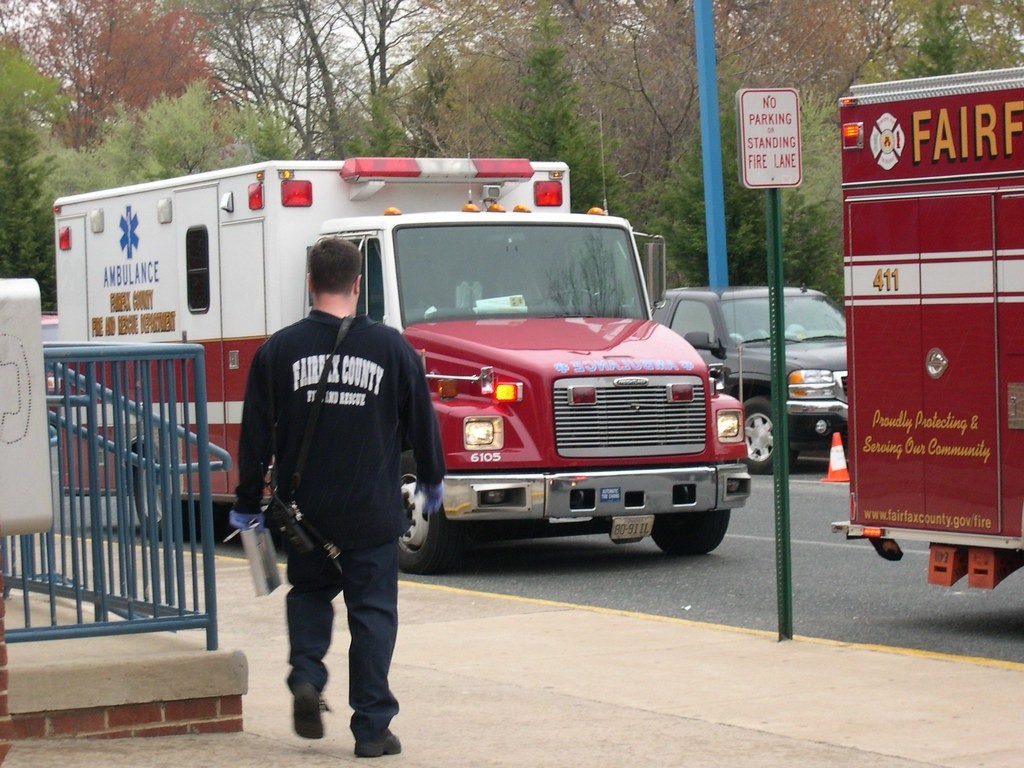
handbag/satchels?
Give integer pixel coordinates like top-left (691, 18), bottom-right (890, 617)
top-left (261, 489), bottom-right (343, 578)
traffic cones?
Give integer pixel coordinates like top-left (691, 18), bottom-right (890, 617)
top-left (817, 433), bottom-right (851, 484)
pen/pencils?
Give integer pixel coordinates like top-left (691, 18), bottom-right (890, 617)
top-left (223, 517), bottom-right (256, 542)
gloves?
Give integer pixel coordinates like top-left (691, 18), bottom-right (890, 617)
top-left (227, 509), bottom-right (266, 535)
top-left (414, 479), bottom-right (444, 522)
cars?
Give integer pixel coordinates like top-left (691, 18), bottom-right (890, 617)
top-left (43, 310), bottom-right (59, 414)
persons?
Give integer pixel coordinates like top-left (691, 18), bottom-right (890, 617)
top-left (230, 236), bottom-right (447, 757)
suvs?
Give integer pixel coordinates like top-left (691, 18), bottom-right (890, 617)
top-left (654, 284), bottom-right (849, 476)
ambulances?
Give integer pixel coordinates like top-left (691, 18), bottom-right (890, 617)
top-left (832, 65), bottom-right (1023, 588)
top-left (43, 147), bottom-right (754, 576)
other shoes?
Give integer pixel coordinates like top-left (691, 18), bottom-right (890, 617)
top-left (353, 728), bottom-right (402, 757)
top-left (293, 682), bottom-right (324, 739)
